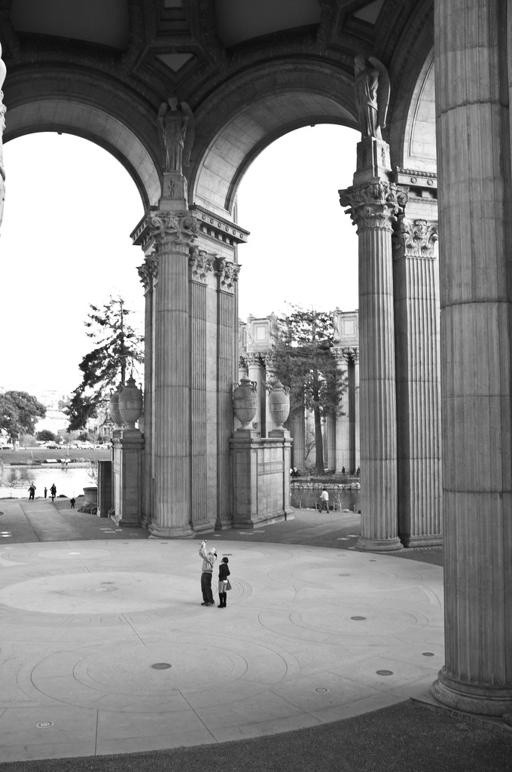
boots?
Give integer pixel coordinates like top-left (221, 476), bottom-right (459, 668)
top-left (218, 591), bottom-right (227, 608)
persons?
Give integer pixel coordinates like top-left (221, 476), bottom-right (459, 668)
top-left (289, 466), bottom-right (360, 514)
top-left (217, 557), bottom-right (230, 608)
top-left (27, 481), bottom-right (76, 509)
top-left (199, 539), bottom-right (217, 607)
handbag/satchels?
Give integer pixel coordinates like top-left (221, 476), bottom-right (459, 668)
top-left (226, 579), bottom-right (232, 590)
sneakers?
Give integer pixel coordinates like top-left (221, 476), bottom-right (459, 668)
top-left (201, 599), bottom-right (214, 606)
